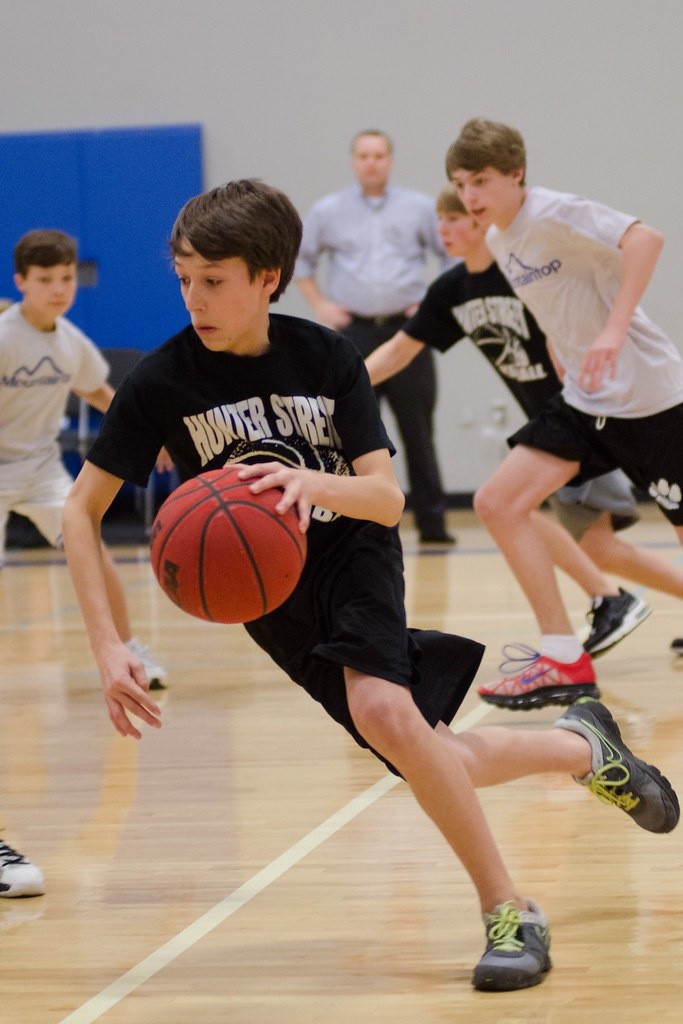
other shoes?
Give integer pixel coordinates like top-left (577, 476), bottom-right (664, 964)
top-left (417, 533), bottom-right (455, 552)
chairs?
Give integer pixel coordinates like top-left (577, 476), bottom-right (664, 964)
top-left (57, 347), bottom-right (181, 531)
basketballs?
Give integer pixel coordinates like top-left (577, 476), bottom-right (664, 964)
top-left (149, 465), bottom-right (308, 626)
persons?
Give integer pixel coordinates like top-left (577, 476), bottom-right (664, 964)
top-left (294, 129), bottom-right (464, 548)
top-left (364, 183), bottom-right (682, 660)
top-left (0, 231), bottom-right (167, 687)
top-left (0, 828), bottom-right (45, 897)
top-left (62, 177), bottom-right (679, 991)
top-left (447, 119), bottom-right (682, 712)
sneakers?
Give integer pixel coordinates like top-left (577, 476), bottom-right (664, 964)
top-left (472, 899), bottom-right (551, 989)
top-left (583, 586), bottom-right (654, 659)
top-left (478, 641), bottom-right (602, 712)
top-left (552, 697), bottom-right (680, 833)
top-left (0, 834), bottom-right (45, 897)
top-left (130, 644), bottom-right (168, 689)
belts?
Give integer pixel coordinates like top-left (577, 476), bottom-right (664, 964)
top-left (352, 312), bottom-right (407, 329)
